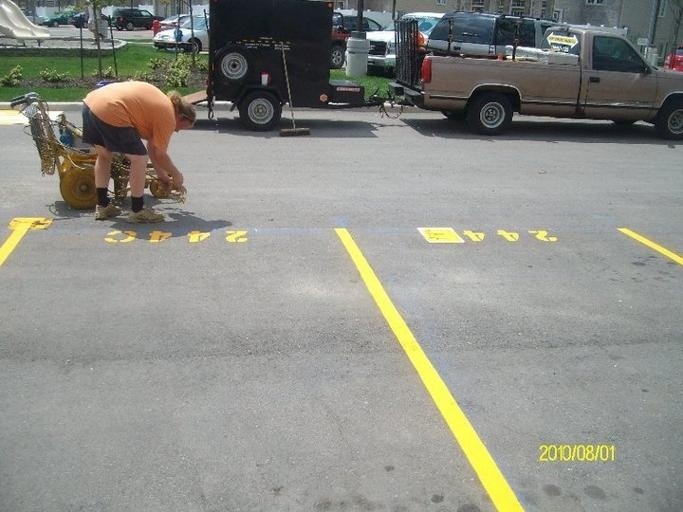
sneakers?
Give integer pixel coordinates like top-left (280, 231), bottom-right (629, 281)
top-left (126, 209), bottom-right (163, 225)
top-left (94, 203), bottom-right (121, 223)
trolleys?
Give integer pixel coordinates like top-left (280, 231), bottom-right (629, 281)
top-left (11, 92), bottom-right (187, 209)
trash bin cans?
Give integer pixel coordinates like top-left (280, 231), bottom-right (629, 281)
top-left (345, 39), bottom-right (369, 76)
top-left (645, 44), bottom-right (657, 66)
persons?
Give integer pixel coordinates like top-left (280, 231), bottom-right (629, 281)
top-left (77, 78), bottom-right (198, 224)
